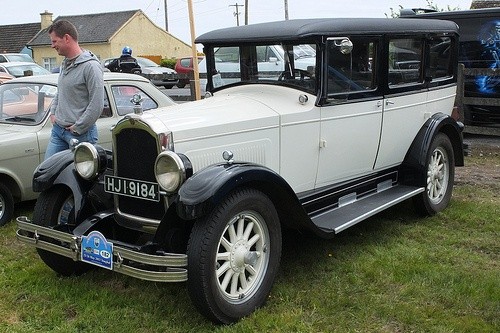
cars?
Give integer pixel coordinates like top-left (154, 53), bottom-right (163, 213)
top-left (0, 71), bottom-right (180, 225)
top-left (104, 56), bottom-right (178, 89)
top-left (194, 31), bottom-right (323, 99)
top-left (1, 53), bottom-right (32, 71)
top-left (0, 72), bottom-right (56, 118)
top-left (1, 62), bottom-right (52, 90)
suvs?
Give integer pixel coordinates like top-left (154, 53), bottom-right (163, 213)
top-left (395, 5), bottom-right (500, 124)
top-left (175, 56), bottom-right (221, 88)
top-left (18, 17), bottom-right (462, 326)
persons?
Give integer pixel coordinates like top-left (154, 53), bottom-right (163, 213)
top-left (16, 18), bottom-right (106, 189)
top-left (103, 45), bottom-right (142, 72)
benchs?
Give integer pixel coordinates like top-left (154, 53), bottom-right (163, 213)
top-left (325, 78), bottom-right (372, 95)
